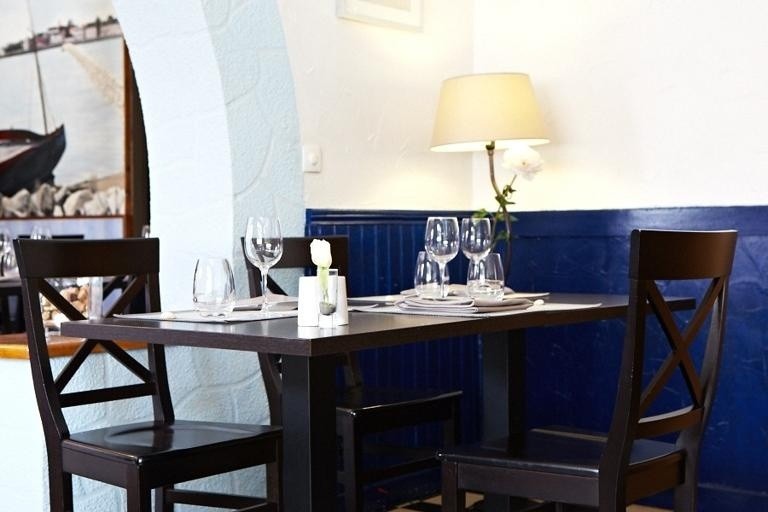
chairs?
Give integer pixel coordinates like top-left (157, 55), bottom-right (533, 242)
top-left (12, 237), bottom-right (285, 512)
top-left (241, 237), bottom-right (462, 512)
top-left (438, 226), bottom-right (739, 512)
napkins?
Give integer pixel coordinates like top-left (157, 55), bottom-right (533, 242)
top-left (394, 296), bottom-right (534, 314)
top-left (232, 294), bottom-right (298, 312)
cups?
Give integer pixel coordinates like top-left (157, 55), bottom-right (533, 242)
top-left (191, 257), bottom-right (236, 319)
top-left (467, 253), bottom-right (505, 302)
top-left (414, 250), bottom-right (450, 300)
top-left (0, 224), bottom-right (49, 277)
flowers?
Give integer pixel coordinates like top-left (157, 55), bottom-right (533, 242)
top-left (310, 239), bottom-right (332, 305)
top-left (471, 143), bottom-right (547, 248)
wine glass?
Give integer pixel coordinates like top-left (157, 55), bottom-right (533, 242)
top-left (460, 218), bottom-right (492, 301)
top-left (243, 216), bottom-right (283, 316)
top-left (423, 217), bottom-right (460, 301)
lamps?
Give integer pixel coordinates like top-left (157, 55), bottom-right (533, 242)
top-left (430, 71), bottom-right (551, 285)
